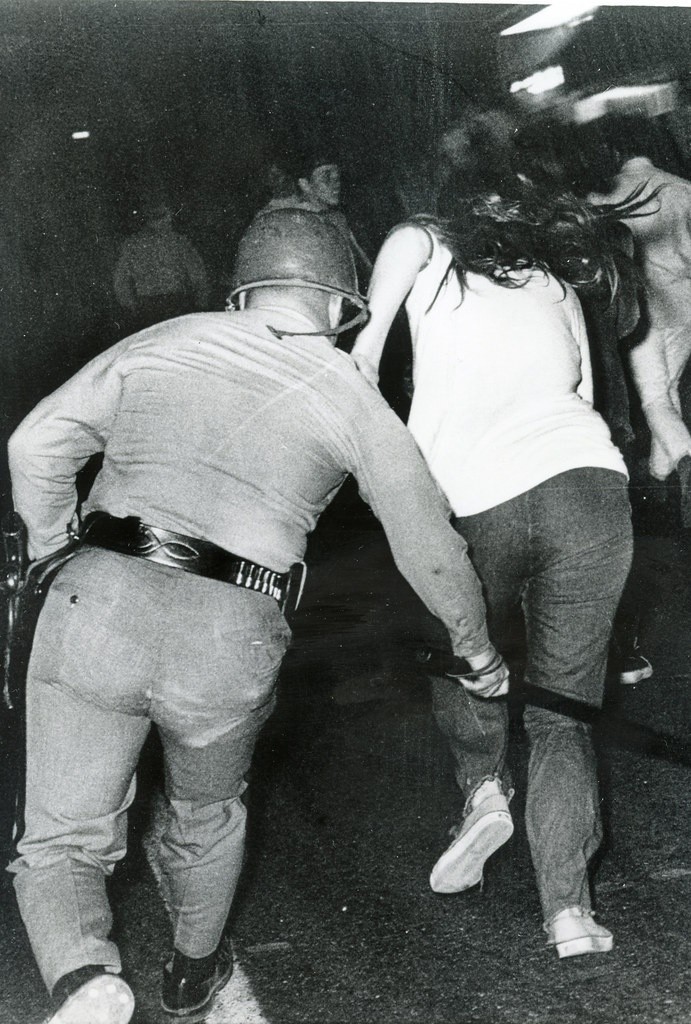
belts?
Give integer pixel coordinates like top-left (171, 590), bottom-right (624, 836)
top-left (78, 510), bottom-right (289, 602)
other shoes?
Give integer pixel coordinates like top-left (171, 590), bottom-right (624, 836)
top-left (430, 790), bottom-right (514, 893)
top-left (549, 911), bottom-right (614, 958)
top-left (618, 654), bottom-right (655, 684)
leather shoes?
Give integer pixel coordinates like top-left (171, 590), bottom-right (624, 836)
top-left (41, 964), bottom-right (135, 1024)
top-left (160, 935), bottom-right (233, 1016)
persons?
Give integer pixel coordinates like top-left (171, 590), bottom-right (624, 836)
top-left (2, 195), bottom-right (525, 1024)
top-left (111, 125), bottom-right (691, 511)
top-left (344, 106), bottom-right (640, 964)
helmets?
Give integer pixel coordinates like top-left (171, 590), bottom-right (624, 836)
top-left (228, 207), bottom-right (365, 310)
